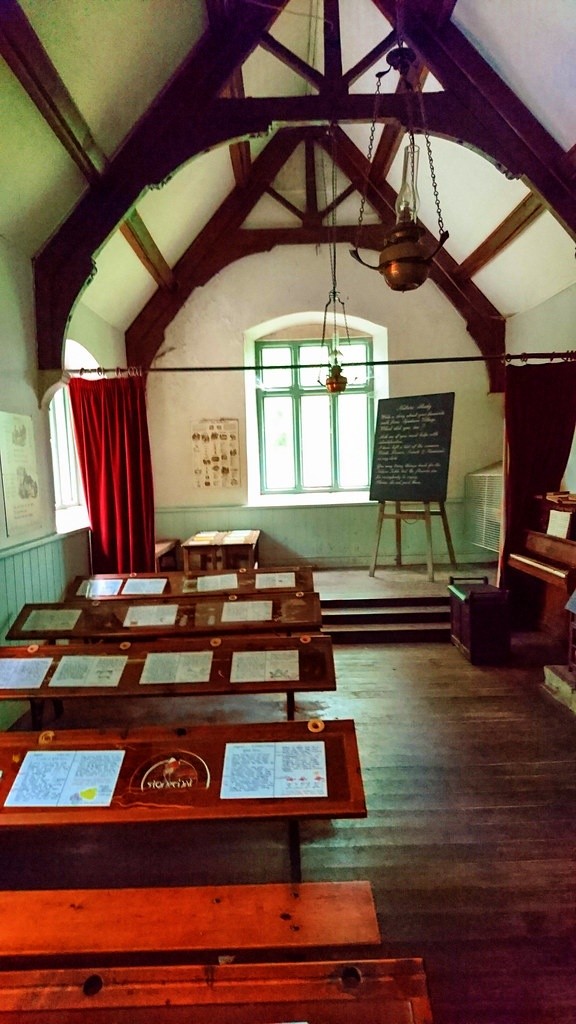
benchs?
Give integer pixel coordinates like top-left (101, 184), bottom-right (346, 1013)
top-left (0, 879), bottom-right (381, 957)
top-left (155, 539), bottom-right (180, 573)
top-left (448, 576), bottom-right (513, 664)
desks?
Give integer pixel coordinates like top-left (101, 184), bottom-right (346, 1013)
top-left (182, 531), bottom-right (227, 571)
top-left (0, 957), bottom-right (433, 1024)
top-left (4, 592), bottom-right (322, 645)
top-left (63, 565), bottom-right (314, 601)
top-left (0, 633), bottom-right (337, 731)
top-left (221, 529), bottom-right (261, 570)
top-left (0, 719), bottom-right (367, 890)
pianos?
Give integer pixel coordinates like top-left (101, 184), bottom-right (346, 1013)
top-left (506, 490), bottom-right (576, 666)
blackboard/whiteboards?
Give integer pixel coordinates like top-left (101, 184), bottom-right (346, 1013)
top-left (370, 392), bottom-right (454, 501)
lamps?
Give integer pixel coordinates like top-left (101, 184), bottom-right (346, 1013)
top-left (317, 130), bottom-right (357, 393)
top-left (350, 0), bottom-right (449, 291)
